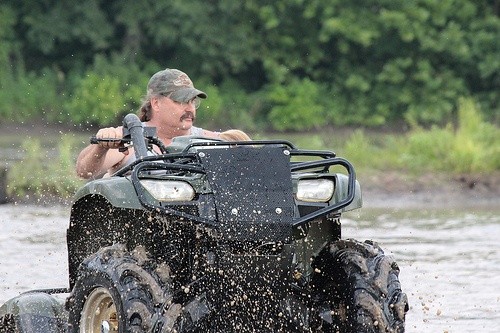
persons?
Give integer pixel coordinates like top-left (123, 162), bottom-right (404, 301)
top-left (76, 68), bottom-right (252, 177)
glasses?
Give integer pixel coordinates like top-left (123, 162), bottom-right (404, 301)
top-left (176, 98), bottom-right (202, 110)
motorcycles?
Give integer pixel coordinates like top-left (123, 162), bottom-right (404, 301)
top-left (0, 113), bottom-right (409, 333)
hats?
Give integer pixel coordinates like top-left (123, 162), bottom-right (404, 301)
top-left (146, 67), bottom-right (207, 102)
